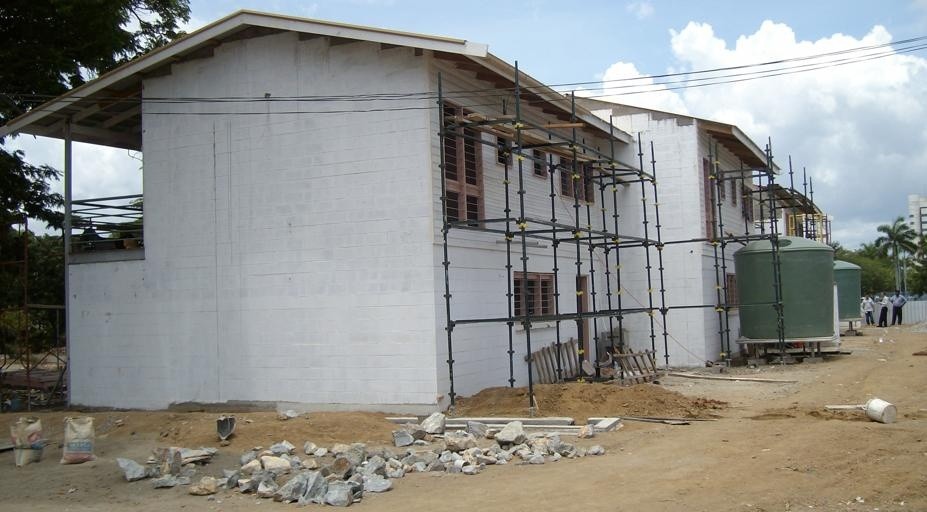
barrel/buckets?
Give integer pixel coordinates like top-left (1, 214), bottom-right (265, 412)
top-left (865, 397), bottom-right (897, 424)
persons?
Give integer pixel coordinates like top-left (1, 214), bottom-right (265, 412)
top-left (860, 294), bottom-right (876, 326)
top-left (890, 289), bottom-right (906, 325)
top-left (876, 292), bottom-right (888, 327)
